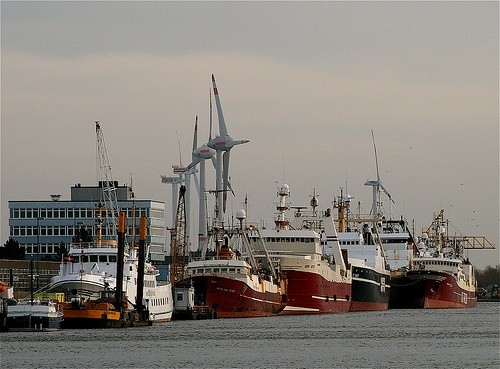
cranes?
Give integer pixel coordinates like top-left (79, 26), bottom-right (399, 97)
top-left (94, 119), bottom-right (129, 244)
top-left (421, 209), bottom-right (496, 249)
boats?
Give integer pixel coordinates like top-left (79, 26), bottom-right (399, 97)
top-left (380, 209), bottom-right (478, 309)
top-left (173, 207), bottom-right (289, 319)
top-left (245, 183), bottom-right (353, 315)
top-left (36, 212), bottom-right (174, 329)
top-left (0, 268), bottom-right (65, 333)
top-left (319, 181), bottom-right (392, 311)
